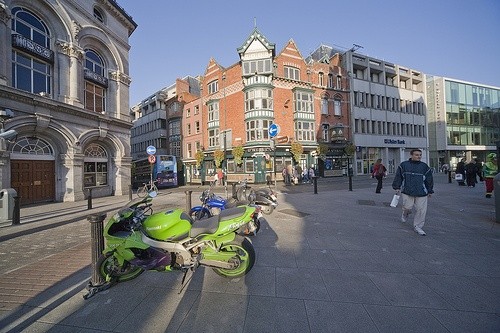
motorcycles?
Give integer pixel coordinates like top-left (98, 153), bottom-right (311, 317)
top-left (226, 181), bottom-right (279, 215)
top-left (97, 181), bottom-right (261, 283)
top-left (188, 180), bottom-right (260, 236)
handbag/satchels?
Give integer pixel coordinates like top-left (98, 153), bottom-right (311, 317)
top-left (454, 174), bottom-right (463, 180)
top-left (390, 191), bottom-right (401, 208)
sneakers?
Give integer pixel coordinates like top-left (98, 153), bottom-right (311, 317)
top-left (401, 211), bottom-right (408, 223)
top-left (414, 226), bottom-right (426, 236)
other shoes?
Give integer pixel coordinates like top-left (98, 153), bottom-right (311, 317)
top-left (376, 192), bottom-right (381, 193)
top-left (486, 194), bottom-right (492, 199)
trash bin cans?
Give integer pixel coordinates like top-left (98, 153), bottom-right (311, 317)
top-left (0, 187), bottom-right (20, 226)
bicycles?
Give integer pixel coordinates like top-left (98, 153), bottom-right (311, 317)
top-left (137, 180), bottom-right (158, 198)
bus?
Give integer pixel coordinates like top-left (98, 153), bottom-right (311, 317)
top-left (131, 155), bottom-right (177, 189)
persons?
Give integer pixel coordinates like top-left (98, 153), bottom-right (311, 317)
top-left (371, 159), bottom-right (387, 193)
top-left (216, 153), bottom-right (498, 198)
top-left (392, 150), bottom-right (434, 236)
